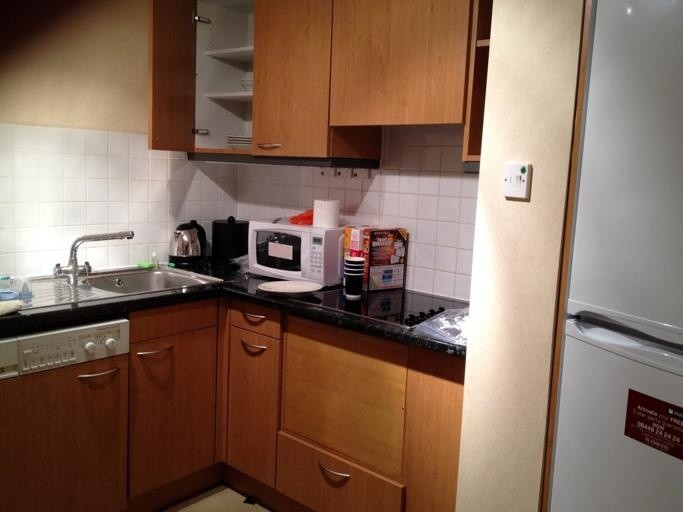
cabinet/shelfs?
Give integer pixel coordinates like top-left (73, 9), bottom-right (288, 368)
top-left (329, 0), bottom-right (469, 127)
top-left (1, 352), bottom-right (129, 512)
top-left (129, 290), bottom-right (223, 511)
top-left (458, 0), bottom-right (491, 166)
top-left (402, 330), bottom-right (469, 512)
top-left (144, 3), bottom-right (381, 171)
top-left (274, 306), bottom-right (411, 511)
top-left (216, 297), bottom-right (288, 490)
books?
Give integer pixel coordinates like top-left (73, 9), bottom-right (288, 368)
top-left (361, 293), bottom-right (404, 325)
top-left (344, 225), bottom-right (407, 294)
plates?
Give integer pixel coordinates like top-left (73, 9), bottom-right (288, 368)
top-left (225, 134), bottom-right (252, 150)
top-left (256, 279), bottom-right (322, 295)
top-left (0, 291), bottom-right (17, 301)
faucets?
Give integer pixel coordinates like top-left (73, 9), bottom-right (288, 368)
top-left (69, 231), bottom-right (134, 285)
top-left (71, 287), bottom-right (79, 310)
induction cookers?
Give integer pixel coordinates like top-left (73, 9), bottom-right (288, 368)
top-left (0, 275), bottom-right (129, 381)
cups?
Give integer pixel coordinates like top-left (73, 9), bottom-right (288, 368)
top-left (344, 255), bottom-right (364, 302)
top-left (11, 275), bottom-right (32, 299)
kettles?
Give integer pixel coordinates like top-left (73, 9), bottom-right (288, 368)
top-left (168, 220), bottom-right (205, 266)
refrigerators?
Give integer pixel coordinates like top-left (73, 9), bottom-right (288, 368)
top-left (533, 1), bottom-right (682, 511)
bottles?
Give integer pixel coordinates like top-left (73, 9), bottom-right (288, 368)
top-left (150, 252), bottom-right (158, 270)
top-left (0, 276), bottom-right (10, 290)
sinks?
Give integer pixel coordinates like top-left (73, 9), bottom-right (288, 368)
top-left (82, 266), bottom-right (224, 294)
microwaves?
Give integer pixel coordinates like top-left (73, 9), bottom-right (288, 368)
top-left (247, 218), bottom-right (346, 289)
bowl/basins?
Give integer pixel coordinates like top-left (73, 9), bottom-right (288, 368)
top-left (240, 78), bottom-right (253, 91)
